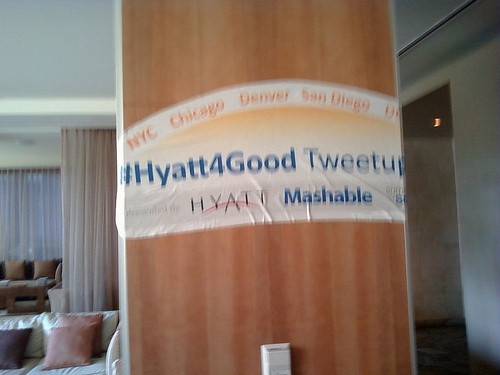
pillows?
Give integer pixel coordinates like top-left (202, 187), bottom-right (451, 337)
top-left (4, 259), bottom-right (27, 280)
top-left (0, 327), bottom-right (33, 370)
top-left (40, 322), bottom-right (98, 370)
top-left (58, 313), bottom-right (103, 358)
top-left (33, 258), bottom-right (56, 280)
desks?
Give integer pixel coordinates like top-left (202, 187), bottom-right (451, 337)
top-left (0, 279), bottom-right (55, 315)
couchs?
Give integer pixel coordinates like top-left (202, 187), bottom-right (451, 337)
top-left (0, 309), bottom-right (119, 375)
top-left (0, 257), bottom-right (63, 301)
